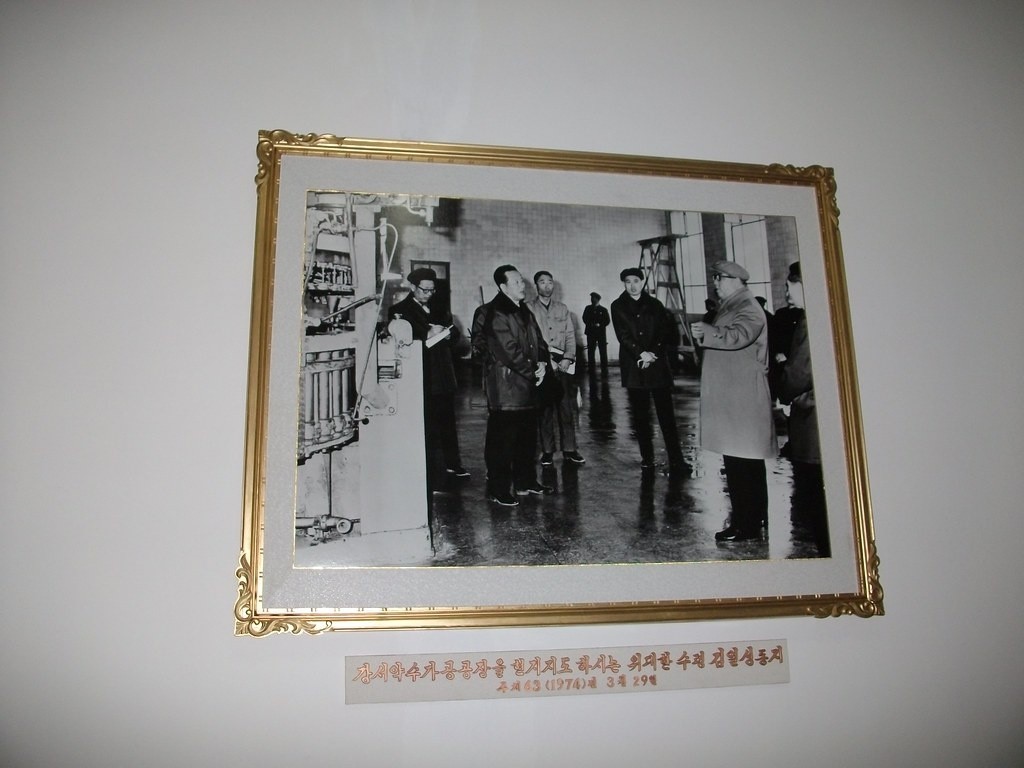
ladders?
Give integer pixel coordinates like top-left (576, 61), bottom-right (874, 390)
top-left (637, 233), bottom-right (699, 365)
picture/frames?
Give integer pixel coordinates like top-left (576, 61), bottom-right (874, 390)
top-left (235, 128), bottom-right (886, 636)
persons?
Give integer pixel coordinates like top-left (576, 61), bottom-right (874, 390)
top-left (391, 253), bottom-right (821, 548)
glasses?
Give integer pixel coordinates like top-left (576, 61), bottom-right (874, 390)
top-left (417, 286), bottom-right (436, 294)
top-left (712, 273), bottom-right (736, 281)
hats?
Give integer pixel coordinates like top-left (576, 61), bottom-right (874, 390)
top-left (704, 299), bottom-right (716, 307)
top-left (755, 296), bottom-right (767, 302)
top-left (407, 268), bottom-right (436, 281)
top-left (714, 262), bottom-right (749, 280)
top-left (590, 292), bottom-right (601, 300)
top-left (620, 268), bottom-right (644, 281)
top-left (789, 263), bottom-right (802, 278)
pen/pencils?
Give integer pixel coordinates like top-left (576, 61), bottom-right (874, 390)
top-left (428, 323), bottom-right (448, 328)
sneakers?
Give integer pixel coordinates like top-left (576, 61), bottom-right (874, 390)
top-left (641, 459), bottom-right (653, 467)
top-left (670, 458), bottom-right (693, 468)
top-left (563, 451), bottom-right (585, 462)
top-left (433, 487), bottom-right (448, 494)
top-left (489, 491), bottom-right (519, 506)
top-left (516, 480), bottom-right (554, 495)
top-left (446, 464), bottom-right (471, 476)
top-left (541, 453), bottom-right (553, 465)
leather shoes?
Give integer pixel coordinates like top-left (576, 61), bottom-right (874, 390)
top-left (715, 526), bottom-right (768, 542)
